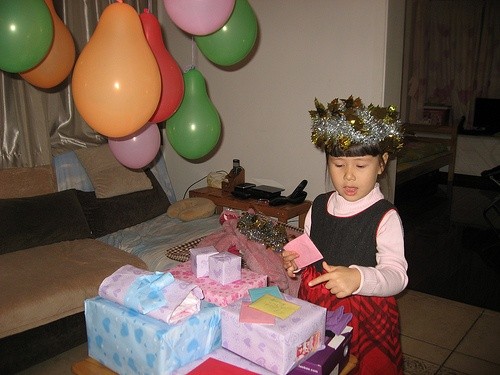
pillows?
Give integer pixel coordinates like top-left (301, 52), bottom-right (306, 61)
top-left (51, 152), bottom-right (95, 192)
top-left (73, 147), bottom-right (153, 198)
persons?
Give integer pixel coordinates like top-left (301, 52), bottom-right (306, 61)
top-left (281, 95), bottom-right (408, 375)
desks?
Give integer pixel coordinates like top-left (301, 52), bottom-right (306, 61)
top-left (189, 186), bottom-right (312, 230)
top-left (71, 354), bottom-right (358, 375)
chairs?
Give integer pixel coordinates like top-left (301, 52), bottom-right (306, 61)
top-left (480, 166), bottom-right (500, 217)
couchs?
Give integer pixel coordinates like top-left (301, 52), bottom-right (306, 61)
top-left (0, 144), bottom-right (224, 375)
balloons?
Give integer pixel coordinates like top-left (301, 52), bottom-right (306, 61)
top-left (108, 122), bottom-right (161, 169)
top-left (0, 0), bottom-right (54, 73)
top-left (166, 68), bottom-right (221, 160)
top-left (163, 0), bottom-right (236, 35)
top-left (138, 8), bottom-right (185, 123)
top-left (193, 0), bottom-right (258, 68)
top-left (19, 0), bottom-right (75, 88)
top-left (71, 0), bottom-right (162, 138)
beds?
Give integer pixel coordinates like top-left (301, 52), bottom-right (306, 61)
top-left (396, 123), bottom-right (460, 187)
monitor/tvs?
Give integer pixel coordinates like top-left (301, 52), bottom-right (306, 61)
top-left (474, 97), bottom-right (500, 132)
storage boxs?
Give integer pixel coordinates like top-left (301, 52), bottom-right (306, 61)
top-left (84, 244), bottom-right (353, 375)
top-left (424, 103), bottom-right (457, 126)
